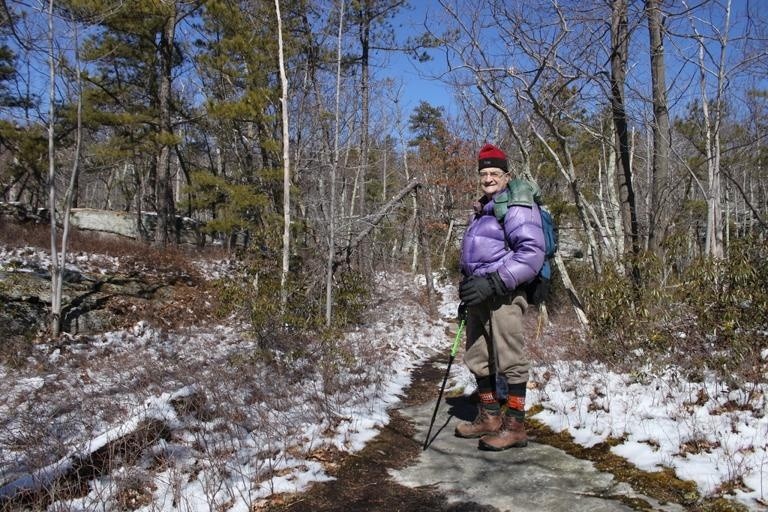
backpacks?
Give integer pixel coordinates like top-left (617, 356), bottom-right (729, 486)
top-left (500, 206), bottom-right (559, 305)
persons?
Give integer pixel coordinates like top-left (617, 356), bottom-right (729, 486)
top-left (454, 142), bottom-right (561, 451)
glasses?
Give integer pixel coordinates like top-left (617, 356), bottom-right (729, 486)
top-left (479, 171), bottom-right (508, 180)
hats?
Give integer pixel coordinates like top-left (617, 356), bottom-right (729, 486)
top-left (477, 143), bottom-right (509, 173)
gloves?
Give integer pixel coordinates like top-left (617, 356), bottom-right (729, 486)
top-left (459, 272), bottom-right (509, 306)
top-left (458, 301), bottom-right (466, 325)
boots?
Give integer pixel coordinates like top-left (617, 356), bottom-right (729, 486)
top-left (479, 415), bottom-right (529, 451)
top-left (455, 404), bottom-right (504, 439)
